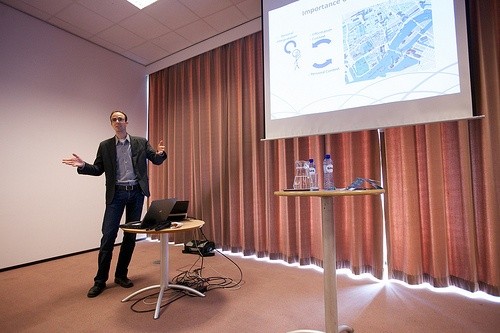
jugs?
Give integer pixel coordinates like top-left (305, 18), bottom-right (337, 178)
top-left (293, 159), bottom-right (312, 189)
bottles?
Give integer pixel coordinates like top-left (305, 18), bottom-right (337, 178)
top-left (323, 154), bottom-right (335, 190)
top-left (308, 158), bottom-right (319, 188)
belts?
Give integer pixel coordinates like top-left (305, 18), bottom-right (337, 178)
top-left (115, 185), bottom-right (141, 190)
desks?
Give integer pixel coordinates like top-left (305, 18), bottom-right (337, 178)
top-left (274, 189), bottom-right (385, 333)
top-left (121, 220), bottom-right (205, 319)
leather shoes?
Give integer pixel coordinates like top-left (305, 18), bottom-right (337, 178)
top-left (114, 277), bottom-right (133, 289)
top-left (88, 282), bottom-right (106, 296)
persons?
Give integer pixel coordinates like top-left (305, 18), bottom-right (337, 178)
top-left (62, 111), bottom-right (167, 297)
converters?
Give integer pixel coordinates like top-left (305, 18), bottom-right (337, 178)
top-left (195, 285), bottom-right (207, 292)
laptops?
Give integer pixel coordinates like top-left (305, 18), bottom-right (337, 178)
top-left (118, 198), bottom-right (177, 229)
